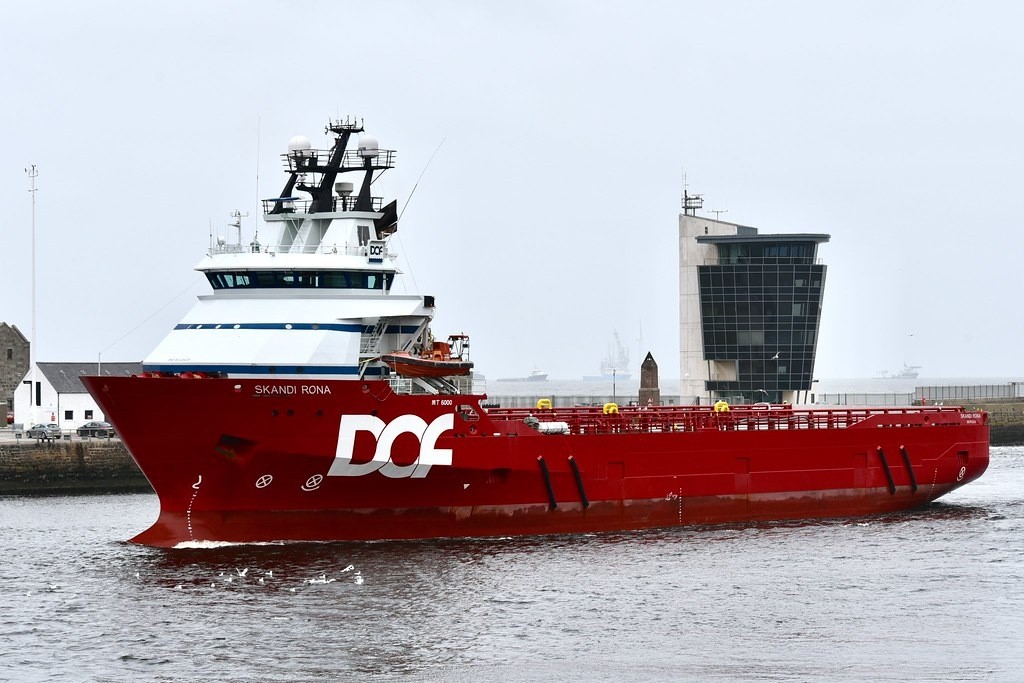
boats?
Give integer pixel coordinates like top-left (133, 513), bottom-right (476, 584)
top-left (497, 369), bottom-right (548, 382)
top-left (891, 362), bottom-right (922, 380)
top-left (381, 333), bottom-right (475, 377)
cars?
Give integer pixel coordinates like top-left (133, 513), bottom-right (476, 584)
top-left (26, 423), bottom-right (62, 440)
top-left (77, 420), bottom-right (115, 437)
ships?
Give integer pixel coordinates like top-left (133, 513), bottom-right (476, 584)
top-left (77, 113), bottom-right (990, 556)
